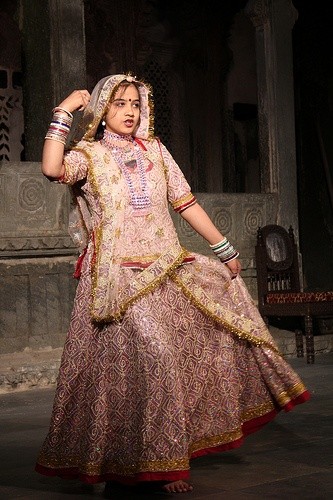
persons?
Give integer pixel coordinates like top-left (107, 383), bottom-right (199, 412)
top-left (34, 75), bottom-right (309, 493)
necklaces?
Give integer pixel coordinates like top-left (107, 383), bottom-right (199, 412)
top-left (100, 129), bottom-right (150, 209)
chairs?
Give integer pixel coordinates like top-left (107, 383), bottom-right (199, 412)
top-left (254, 224), bottom-right (333, 365)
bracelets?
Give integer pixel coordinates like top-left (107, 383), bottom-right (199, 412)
top-left (209, 236), bottom-right (239, 264)
top-left (45, 107), bottom-right (73, 146)
